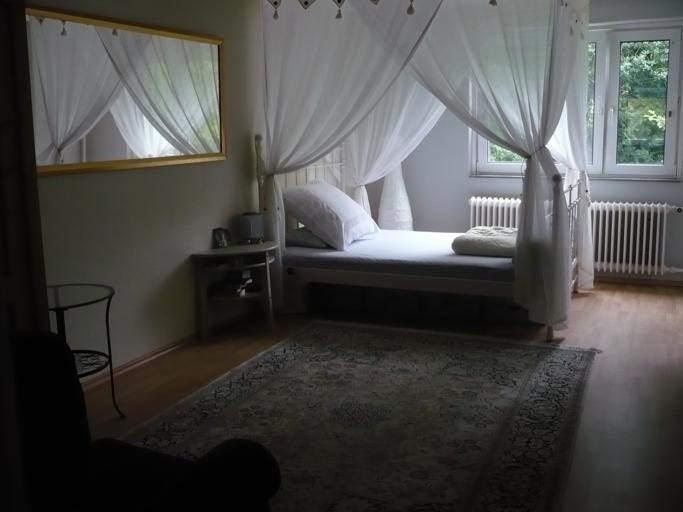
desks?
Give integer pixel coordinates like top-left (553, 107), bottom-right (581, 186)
top-left (45, 281), bottom-right (126, 425)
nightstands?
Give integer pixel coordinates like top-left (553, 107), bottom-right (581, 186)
top-left (189, 241), bottom-right (276, 336)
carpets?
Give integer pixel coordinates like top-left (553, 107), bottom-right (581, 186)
top-left (116, 319), bottom-right (593, 512)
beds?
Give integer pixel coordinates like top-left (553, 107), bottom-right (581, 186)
top-left (253, 133), bottom-right (586, 343)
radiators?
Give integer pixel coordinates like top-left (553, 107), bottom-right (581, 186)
top-left (468, 196), bottom-right (682, 277)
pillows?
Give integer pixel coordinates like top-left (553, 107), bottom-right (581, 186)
top-left (284, 226), bottom-right (326, 247)
top-left (282, 178), bottom-right (381, 251)
top-left (450, 226), bottom-right (518, 257)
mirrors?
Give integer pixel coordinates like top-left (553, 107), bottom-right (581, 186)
top-left (24, 6), bottom-right (227, 176)
